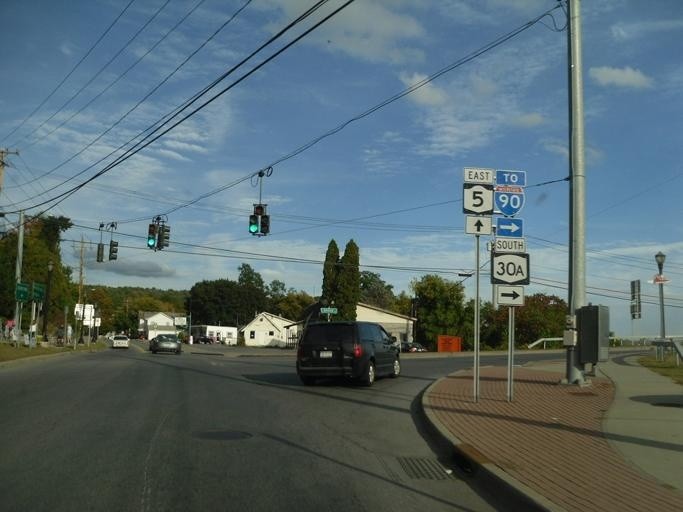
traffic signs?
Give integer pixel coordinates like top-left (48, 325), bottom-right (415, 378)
top-left (463, 167), bottom-right (530, 307)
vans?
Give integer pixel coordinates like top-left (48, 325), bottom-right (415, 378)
top-left (297, 322), bottom-right (401, 386)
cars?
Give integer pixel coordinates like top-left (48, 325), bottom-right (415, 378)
top-left (112, 335), bottom-right (130, 348)
top-left (149, 334), bottom-right (182, 354)
top-left (398, 343), bottom-right (424, 353)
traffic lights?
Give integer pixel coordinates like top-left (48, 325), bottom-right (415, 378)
top-left (249, 204), bottom-right (270, 236)
top-left (109, 241), bottom-right (118, 261)
top-left (159, 225), bottom-right (170, 250)
top-left (147, 224), bottom-right (157, 249)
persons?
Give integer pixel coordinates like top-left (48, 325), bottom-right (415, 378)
top-left (41, 329), bottom-right (49, 343)
top-left (52, 323), bottom-right (64, 347)
top-left (4, 313), bottom-right (16, 347)
top-left (27, 319), bottom-right (37, 343)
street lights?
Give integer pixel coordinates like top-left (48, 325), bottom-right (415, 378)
top-left (42, 260), bottom-right (55, 335)
top-left (79, 285), bottom-right (96, 340)
top-left (656, 252), bottom-right (666, 337)
top-left (328, 300), bottom-right (336, 321)
